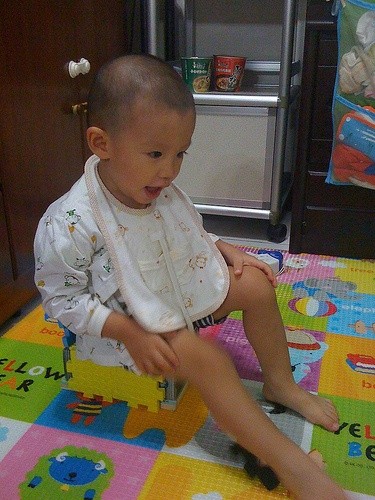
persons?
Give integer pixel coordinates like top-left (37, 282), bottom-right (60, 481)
top-left (31, 54), bottom-right (354, 500)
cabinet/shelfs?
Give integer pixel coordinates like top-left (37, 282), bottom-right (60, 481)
top-left (0, 1), bottom-right (154, 319)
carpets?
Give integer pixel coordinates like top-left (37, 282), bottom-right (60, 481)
top-left (0, 242), bottom-right (375, 500)
